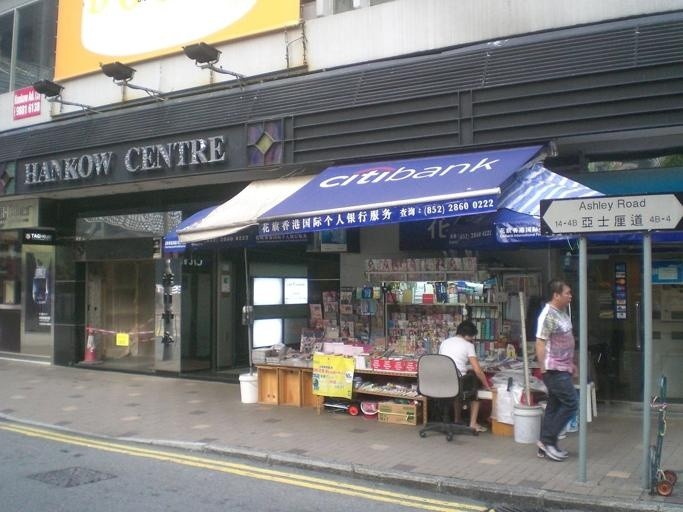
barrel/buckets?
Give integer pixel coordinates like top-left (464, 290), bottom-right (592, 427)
top-left (507, 343), bottom-right (516, 358)
top-left (513, 404), bottom-right (544, 444)
top-left (238, 373), bottom-right (259, 403)
top-left (360, 400), bottom-right (379, 419)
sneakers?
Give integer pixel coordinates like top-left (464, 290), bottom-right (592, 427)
top-left (470, 424), bottom-right (488, 432)
top-left (537, 441), bottom-right (569, 460)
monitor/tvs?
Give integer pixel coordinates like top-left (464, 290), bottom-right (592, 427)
top-left (284, 277), bottom-right (310, 306)
top-left (251, 276), bottom-right (283, 307)
top-left (283, 316), bottom-right (309, 346)
top-left (252, 317), bottom-right (283, 349)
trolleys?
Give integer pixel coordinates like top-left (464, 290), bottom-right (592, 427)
top-left (649, 376), bottom-right (676, 496)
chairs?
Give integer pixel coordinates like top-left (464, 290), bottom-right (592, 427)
top-left (416, 353), bottom-right (478, 443)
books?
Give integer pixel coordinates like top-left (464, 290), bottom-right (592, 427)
top-left (251, 257), bottom-right (524, 372)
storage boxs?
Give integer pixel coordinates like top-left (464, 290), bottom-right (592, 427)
top-left (378, 400), bottom-right (421, 426)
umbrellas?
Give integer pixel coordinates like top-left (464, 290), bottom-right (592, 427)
top-left (448, 208), bottom-right (581, 280)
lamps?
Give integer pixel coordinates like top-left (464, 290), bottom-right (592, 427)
top-left (31, 42), bottom-right (243, 111)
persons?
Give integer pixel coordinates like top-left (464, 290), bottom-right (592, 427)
top-left (439, 320), bottom-right (491, 433)
top-left (535, 278), bottom-right (579, 461)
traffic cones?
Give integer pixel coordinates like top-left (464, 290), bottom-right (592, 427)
top-left (79, 322), bottom-right (103, 366)
